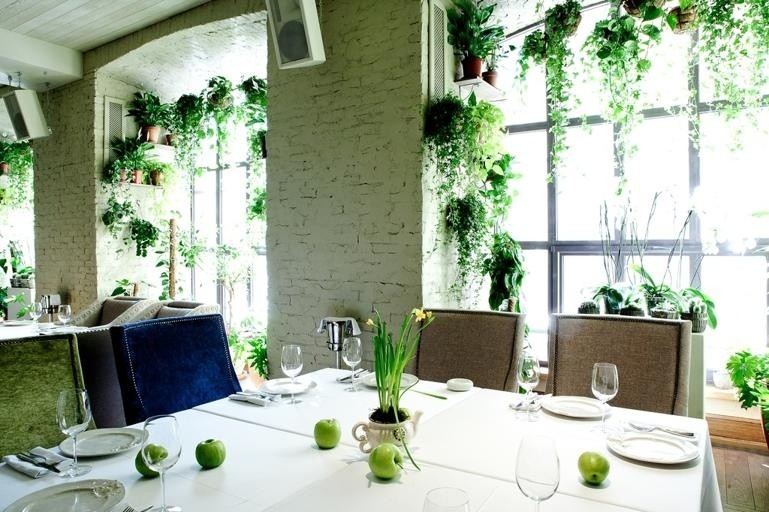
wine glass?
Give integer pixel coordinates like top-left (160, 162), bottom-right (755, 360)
top-left (342, 337), bottom-right (366, 393)
top-left (590, 362), bottom-right (620, 434)
top-left (138, 414), bottom-right (183, 512)
top-left (30, 302), bottom-right (43, 329)
top-left (514, 354), bottom-right (542, 419)
top-left (56, 385), bottom-right (93, 480)
top-left (58, 305), bottom-right (72, 329)
top-left (280, 344), bottom-right (308, 406)
top-left (513, 446), bottom-right (563, 512)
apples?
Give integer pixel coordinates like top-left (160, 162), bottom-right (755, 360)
top-left (195, 438), bottom-right (226, 468)
top-left (134, 445), bottom-right (167, 476)
top-left (313, 418), bottom-right (340, 449)
top-left (579, 451), bottom-right (609, 484)
top-left (368, 443), bottom-right (404, 480)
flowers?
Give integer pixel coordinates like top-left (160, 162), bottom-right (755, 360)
top-left (358, 302), bottom-right (435, 471)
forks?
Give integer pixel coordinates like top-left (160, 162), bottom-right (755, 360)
top-left (631, 422), bottom-right (697, 438)
top-left (123, 505), bottom-right (134, 512)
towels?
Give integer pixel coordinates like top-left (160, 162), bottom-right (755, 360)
top-left (3, 444), bottom-right (74, 479)
top-left (623, 419), bottom-right (702, 446)
top-left (227, 389), bottom-right (282, 408)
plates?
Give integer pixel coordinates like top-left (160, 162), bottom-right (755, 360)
top-left (58, 426), bottom-right (148, 457)
top-left (259, 379), bottom-right (318, 396)
top-left (605, 431), bottom-right (700, 467)
top-left (0, 479), bottom-right (127, 512)
top-left (362, 372), bottom-right (419, 390)
top-left (540, 393), bottom-right (611, 421)
top-left (1, 321), bottom-right (32, 327)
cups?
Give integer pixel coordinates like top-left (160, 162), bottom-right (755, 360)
top-left (421, 486), bottom-right (472, 511)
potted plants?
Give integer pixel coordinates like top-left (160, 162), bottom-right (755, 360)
top-left (0, 138), bottom-right (35, 209)
top-left (224, 321), bottom-right (267, 391)
top-left (728, 354), bottom-right (769, 448)
top-left (10, 267), bottom-right (35, 290)
top-left (103, 74), bottom-right (267, 255)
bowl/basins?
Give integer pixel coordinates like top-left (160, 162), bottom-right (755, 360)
top-left (447, 378), bottom-right (474, 392)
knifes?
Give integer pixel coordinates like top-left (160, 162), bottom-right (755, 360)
top-left (339, 367), bottom-right (367, 380)
top-left (17, 452), bottom-right (61, 475)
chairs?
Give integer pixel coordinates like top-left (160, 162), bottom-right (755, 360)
top-left (418, 306), bottom-right (527, 390)
top-left (1, 333), bottom-right (98, 457)
top-left (546, 312), bottom-right (693, 416)
top-left (39, 292), bottom-right (152, 334)
top-left (110, 312), bottom-right (245, 422)
top-left (39, 299), bottom-right (221, 429)
